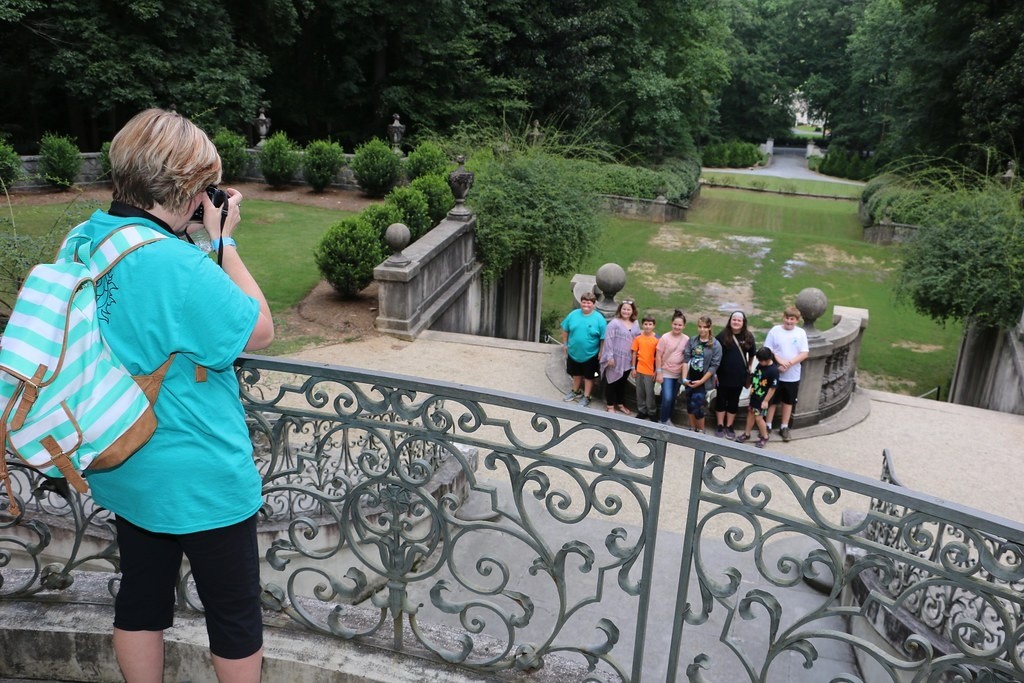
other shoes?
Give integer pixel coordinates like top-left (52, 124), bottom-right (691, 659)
top-left (607, 405), bottom-right (615, 413)
top-left (618, 404), bottom-right (630, 414)
top-left (696, 428), bottom-right (705, 433)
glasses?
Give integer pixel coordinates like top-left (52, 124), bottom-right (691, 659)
top-left (622, 299), bottom-right (633, 305)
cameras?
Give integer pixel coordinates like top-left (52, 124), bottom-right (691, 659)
top-left (187, 184), bottom-right (230, 223)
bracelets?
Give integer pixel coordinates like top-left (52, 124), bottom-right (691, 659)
top-left (682, 378), bottom-right (687, 383)
top-left (631, 366), bottom-right (635, 369)
top-left (713, 374), bottom-right (717, 377)
top-left (212, 237), bottom-right (235, 252)
top-left (656, 369), bottom-right (661, 372)
top-left (564, 342), bottom-right (567, 346)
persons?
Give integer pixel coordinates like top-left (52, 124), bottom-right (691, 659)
top-left (758, 306), bottom-right (810, 441)
top-left (560, 292), bottom-right (607, 407)
top-left (630, 314), bottom-right (661, 423)
top-left (599, 298), bottom-right (640, 415)
top-left (66, 108), bottom-right (274, 683)
top-left (715, 310), bottom-right (757, 439)
top-left (737, 347), bottom-right (780, 448)
top-left (654, 310), bottom-right (690, 426)
top-left (681, 316), bottom-right (722, 435)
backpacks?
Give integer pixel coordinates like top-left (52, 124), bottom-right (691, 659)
top-left (0, 220), bottom-right (206, 516)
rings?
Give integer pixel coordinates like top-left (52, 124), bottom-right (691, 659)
top-left (238, 203), bottom-right (241, 207)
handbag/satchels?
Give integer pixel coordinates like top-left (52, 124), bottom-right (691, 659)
top-left (741, 369), bottom-right (755, 389)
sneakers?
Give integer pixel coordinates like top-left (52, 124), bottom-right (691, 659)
top-left (757, 434), bottom-right (769, 447)
top-left (758, 425), bottom-right (772, 438)
top-left (563, 387), bottom-right (583, 402)
top-left (735, 433), bottom-right (751, 442)
top-left (575, 394), bottom-right (592, 407)
top-left (779, 427), bottom-right (792, 441)
top-left (715, 425), bottom-right (725, 439)
top-left (724, 425), bottom-right (736, 439)
